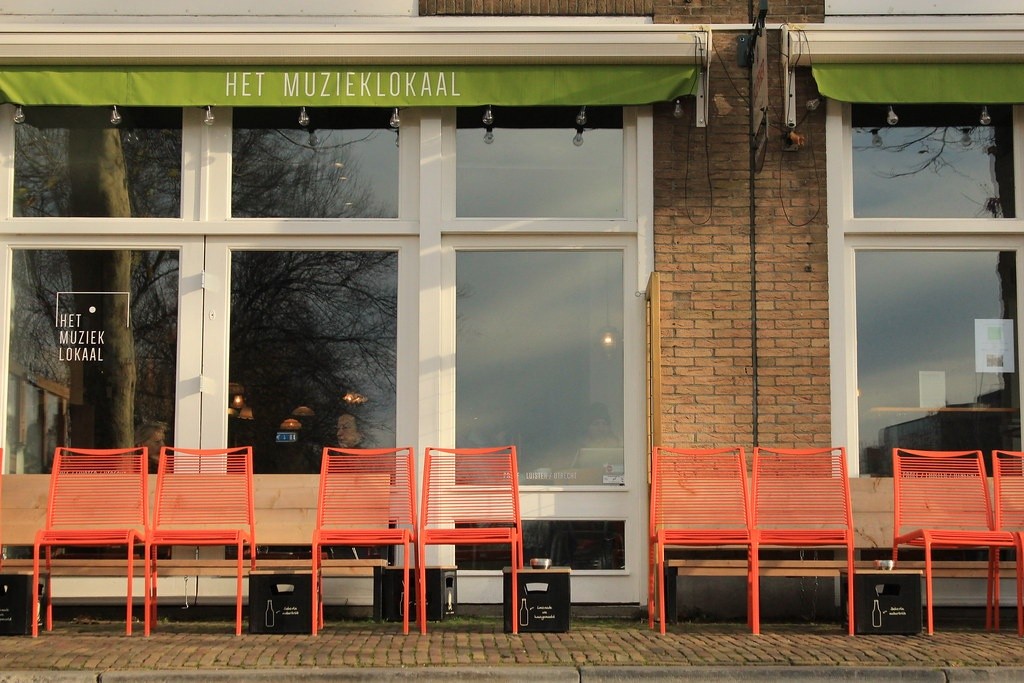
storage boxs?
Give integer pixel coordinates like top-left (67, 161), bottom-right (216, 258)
top-left (246, 569), bottom-right (313, 636)
top-left (836, 568), bottom-right (924, 637)
top-left (385, 567), bottom-right (458, 620)
top-left (501, 564), bottom-right (571, 634)
top-left (0, 566), bottom-right (46, 637)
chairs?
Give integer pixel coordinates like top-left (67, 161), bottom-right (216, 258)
top-left (415, 444), bottom-right (526, 638)
top-left (749, 444), bottom-right (856, 638)
top-left (143, 443), bottom-right (257, 638)
top-left (991, 448), bottom-right (1024, 634)
top-left (311, 446), bottom-right (421, 636)
top-left (889, 444), bottom-right (1021, 636)
top-left (34, 445), bottom-right (152, 635)
top-left (647, 443), bottom-right (753, 638)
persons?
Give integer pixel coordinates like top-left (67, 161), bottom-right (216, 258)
top-left (574, 403), bottom-right (620, 449)
top-left (336, 413), bottom-right (373, 448)
top-left (133, 423), bottom-right (167, 474)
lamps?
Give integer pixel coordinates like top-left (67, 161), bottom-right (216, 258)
top-left (110, 103), bottom-right (121, 125)
top-left (227, 407), bottom-right (237, 418)
top-left (867, 127), bottom-right (885, 147)
top-left (126, 129), bottom-right (138, 145)
top-left (233, 392), bottom-right (249, 408)
top-left (202, 104), bottom-right (216, 127)
top-left (978, 103), bottom-right (995, 125)
top-left (572, 128), bottom-right (587, 146)
top-left (14, 103), bottom-right (26, 124)
top-left (576, 105), bottom-right (588, 126)
top-left (343, 390), bottom-right (368, 408)
top-left (959, 125), bottom-right (978, 149)
top-left (394, 130), bottom-right (401, 147)
top-left (482, 129), bottom-right (496, 146)
top-left (389, 107), bottom-right (402, 129)
top-left (291, 405), bottom-right (315, 419)
top-left (307, 128), bottom-right (320, 147)
top-left (483, 105), bottom-right (495, 127)
top-left (239, 406), bottom-right (254, 420)
top-left (674, 96), bottom-right (684, 120)
top-left (298, 106), bottom-right (310, 125)
top-left (806, 94), bottom-right (826, 112)
top-left (281, 420), bottom-right (303, 433)
top-left (598, 325), bottom-right (618, 355)
top-left (885, 104), bottom-right (901, 125)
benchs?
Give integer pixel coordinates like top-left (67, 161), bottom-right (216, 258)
top-left (0, 473), bottom-right (396, 625)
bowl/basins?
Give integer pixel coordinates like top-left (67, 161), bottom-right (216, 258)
top-left (873, 560), bottom-right (895, 570)
top-left (531, 558), bottom-right (552, 570)
